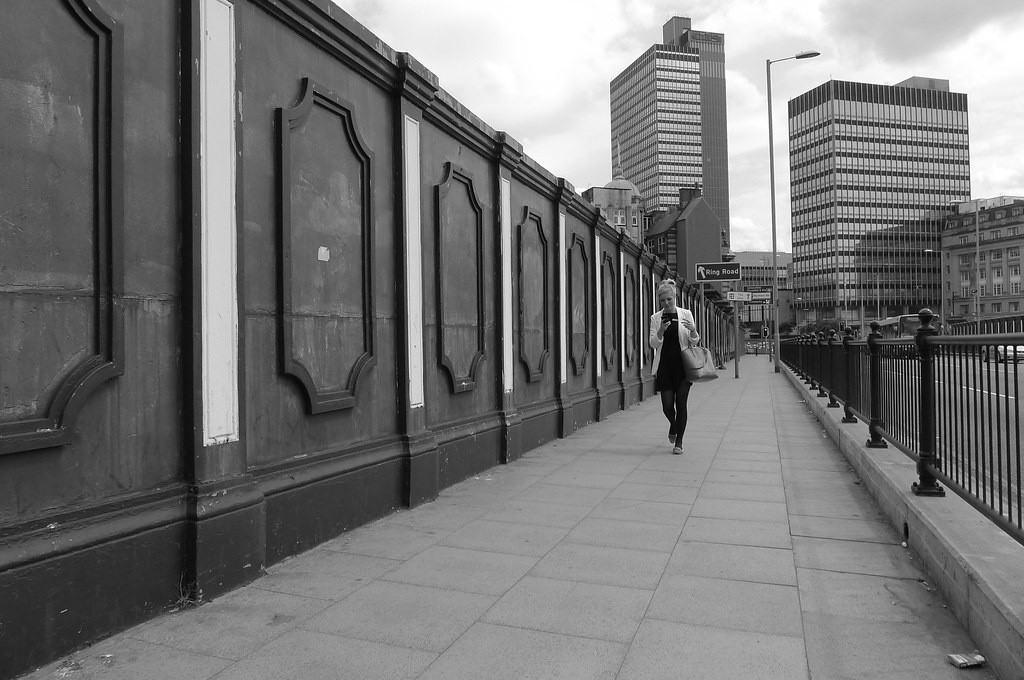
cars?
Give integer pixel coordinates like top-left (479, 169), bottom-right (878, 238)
top-left (982, 346), bottom-right (1024, 363)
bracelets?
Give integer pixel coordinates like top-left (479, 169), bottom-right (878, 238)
top-left (691, 329), bottom-right (695, 332)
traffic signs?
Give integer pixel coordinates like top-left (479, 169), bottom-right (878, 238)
top-left (696, 262), bottom-right (743, 281)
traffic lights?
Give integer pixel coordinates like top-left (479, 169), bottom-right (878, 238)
top-left (839, 322), bottom-right (843, 332)
top-left (764, 327), bottom-right (768, 337)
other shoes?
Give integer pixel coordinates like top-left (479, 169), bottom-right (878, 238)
top-left (673, 444), bottom-right (684, 454)
top-left (669, 430), bottom-right (676, 443)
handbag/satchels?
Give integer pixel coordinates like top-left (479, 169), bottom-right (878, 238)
top-left (682, 336), bottom-right (720, 383)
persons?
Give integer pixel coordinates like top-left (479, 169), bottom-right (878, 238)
top-left (650, 280), bottom-right (699, 455)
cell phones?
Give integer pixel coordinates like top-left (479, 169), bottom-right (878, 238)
top-left (661, 316), bottom-right (669, 323)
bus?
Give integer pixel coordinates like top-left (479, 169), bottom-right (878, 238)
top-left (869, 314), bottom-right (943, 359)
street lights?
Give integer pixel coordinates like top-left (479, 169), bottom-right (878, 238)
top-left (797, 298), bottom-right (810, 329)
top-left (925, 249), bottom-right (944, 334)
top-left (766, 50), bottom-right (821, 373)
top-left (766, 255), bottom-right (781, 355)
top-left (950, 200), bottom-right (980, 334)
